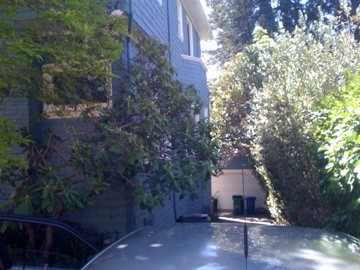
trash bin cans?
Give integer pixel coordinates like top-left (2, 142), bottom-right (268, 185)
top-left (245, 197), bottom-right (257, 215)
top-left (232, 194), bottom-right (244, 216)
top-left (211, 198), bottom-right (218, 213)
